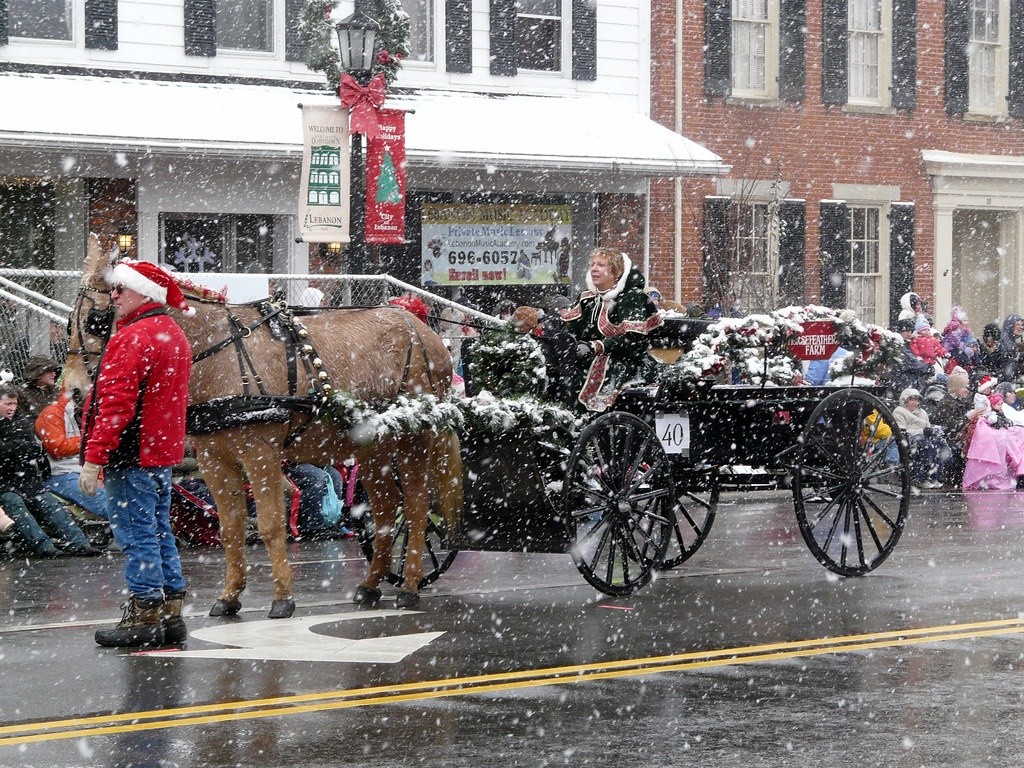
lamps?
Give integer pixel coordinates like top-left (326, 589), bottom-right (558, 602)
top-left (112, 222), bottom-right (133, 253)
top-left (324, 241), bottom-right (341, 258)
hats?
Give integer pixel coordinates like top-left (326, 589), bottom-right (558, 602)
top-left (550, 297), bottom-right (570, 311)
top-left (947, 366), bottom-right (969, 393)
top-left (580, 291), bottom-right (596, 303)
top-left (910, 295), bottom-right (922, 307)
top-left (648, 292), bottom-right (659, 298)
top-left (896, 319), bottom-right (914, 332)
top-left (643, 287), bottom-right (661, 296)
top-left (105, 257), bottom-right (196, 317)
top-left (984, 324), bottom-right (1001, 340)
top-left (915, 314), bottom-right (930, 332)
top-left (974, 376), bottom-right (1014, 414)
top-left (961, 335), bottom-right (980, 351)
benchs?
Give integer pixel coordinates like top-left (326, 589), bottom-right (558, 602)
top-left (642, 316), bottom-right (734, 385)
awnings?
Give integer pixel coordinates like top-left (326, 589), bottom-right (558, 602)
top-left (0, 62), bottom-right (733, 178)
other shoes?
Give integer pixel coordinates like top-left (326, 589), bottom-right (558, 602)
top-left (0, 508), bottom-right (16, 532)
top-left (922, 480), bottom-right (943, 490)
top-left (67, 539), bottom-right (102, 557)
top-left (342, 527), bottom-right (354, 538)
top-left (33, 547), bottom-right (64, 559)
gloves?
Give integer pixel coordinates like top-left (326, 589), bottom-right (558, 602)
top-left (963, 347), bottom-right (974, 358)
top-left (576, 344), bottom-right (591, 359)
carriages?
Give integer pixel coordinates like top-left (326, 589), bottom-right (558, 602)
top-left (61, 247), bottom-right (918, 599)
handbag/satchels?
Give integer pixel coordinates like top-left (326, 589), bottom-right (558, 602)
top-left (80, 413), bottom-right (141, 471)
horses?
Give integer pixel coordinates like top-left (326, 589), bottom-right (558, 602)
top-left (65, 231), bottom-right (464, 619)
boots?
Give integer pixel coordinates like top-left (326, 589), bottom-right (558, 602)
top-left (95, 596), bottom-right (165, 647)
top-left (165, 590), bottom-right (187, 642)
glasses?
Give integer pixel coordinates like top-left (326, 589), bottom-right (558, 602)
top-left (537, 318), bottom-right (545, 323)
top-left (112, 286), bottom-right (124, 294)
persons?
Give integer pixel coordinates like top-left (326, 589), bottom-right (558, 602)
top-left (516, 251), bottom-right (530, 278)
top-left (802, 291), bottom-right (1024, 488)
top-left (557, 237), bottom-right (569, 278)
top-left (78, 260), bottom-right (192, 647)
top-left (541, 245), bottom-right (659, 419)
top-left (436, 285), bottom-right (787, 475)
top-left (0, 286), bottom-right (360, 558)
top-left (422, 259), bottom-right (437, 285)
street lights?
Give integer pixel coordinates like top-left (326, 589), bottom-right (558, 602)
top-left (331, 1), bottom-right (387, 307)
